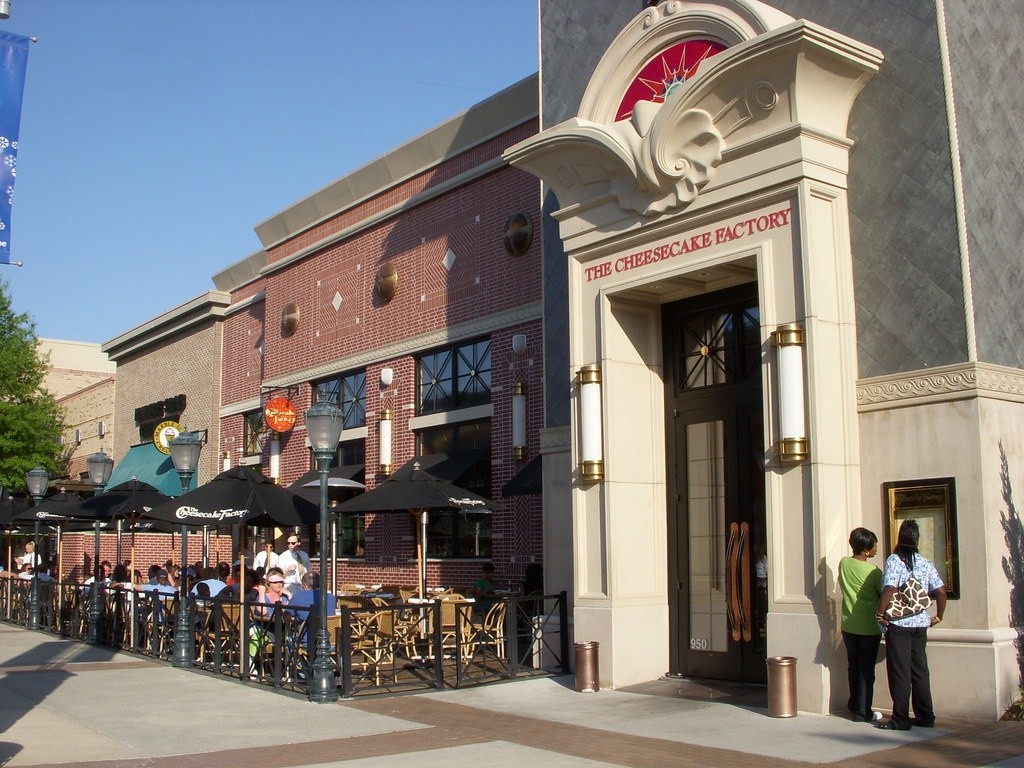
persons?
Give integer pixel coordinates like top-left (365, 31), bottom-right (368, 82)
top-left (12, 541), bottom-right (59, 628)
top-left (875, 517), bottom-right (947, 731)
top-left (79, 533), bottom-right (337, 683)
top-left (837, 527), bottom-right (883, 723)
top-left (471, 561), bottom-right (503, 624)
top-left (523, 562), bottom-right (544, 617)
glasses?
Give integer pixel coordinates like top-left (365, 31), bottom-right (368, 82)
top-left (286, 541), bottom-right (298, 545)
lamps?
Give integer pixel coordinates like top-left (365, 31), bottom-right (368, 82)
top-left (270, 431), bottom-right (279, 484)
top-left (575, 365), bottom-right (604, 480)
top-left (98, 420), bottom-right (106, 436)
top-left (223, 451), bottom-right (231, 471)
top-left (769, 324), bottom-right (809, 461)
top-left (380, 408), bottom-right (391, 476)
top-left (510, 383), bottom-right (528, 462)
top-left (75, 428), bottom-right (82, 443)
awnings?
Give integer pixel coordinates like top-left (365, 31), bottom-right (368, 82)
top-left (500, 453), bottom-right (541, 497)
top-left (103, 431), bottom-right (199, 496)
top-left (285, 464), bottom-right (365, 492)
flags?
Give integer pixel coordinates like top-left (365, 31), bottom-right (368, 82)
top-left (381, 451), bottom-right (490, 488)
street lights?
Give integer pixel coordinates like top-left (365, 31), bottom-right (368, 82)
top-left (87, 445), bottom-right (115, 645)
top-left (26, 463), bottom-right (49, 631)
top-left (302, 388), bottom-right (347, 705)
top-left (168, 423), bottom-right (204, 669)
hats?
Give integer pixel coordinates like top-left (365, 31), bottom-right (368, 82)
top-left (268, 575), bottom-right (284, 582)
top-left (156, 569), bottom-right (168, 578)
top-left (482, 562), bottom-right (498, 572)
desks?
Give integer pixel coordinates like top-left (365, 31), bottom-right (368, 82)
top-left (255, 609), bottom-right (373, 684)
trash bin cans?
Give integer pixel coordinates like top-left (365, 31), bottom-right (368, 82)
top-left (531, 615), bottom-right (561, 670)
top-left (766, 656), bottom-right (798, 717)
top-left (575, 641), bottom-right (601, 693)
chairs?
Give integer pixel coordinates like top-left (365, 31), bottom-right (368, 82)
top-left (0, 563), bottom-right (544, 687)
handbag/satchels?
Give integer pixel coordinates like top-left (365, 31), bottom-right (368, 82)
top-left (883, 576), bottom-right (933, 620)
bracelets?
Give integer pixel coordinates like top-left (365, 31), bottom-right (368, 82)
top-left (935, 616), bottom-right (940, 623)
top-left (875, 610), bottom-right (885, 622)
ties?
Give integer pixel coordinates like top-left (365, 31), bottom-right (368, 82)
top-left (263, 554), bottom-right (267, 573)
top-left (295, 552), bottom-right (305, 580)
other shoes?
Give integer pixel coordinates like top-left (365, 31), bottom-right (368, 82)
top-left (854, 711), bottom-right (883, 721)
top-left (910, 718), bottom-right (934, 727)
top-left (879, 720), bottom-right (911, 730)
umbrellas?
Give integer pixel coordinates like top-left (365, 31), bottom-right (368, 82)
top-left (136, 457), bottom-right (340, 682)
top-left (47, 478), bottom-right (182, 647)
top-left (327, 460), bottom-right (508, 664)
top-left (11, 487), bottom-right (95, 631)
top-left (0, 494), bottom-right (34, 623)
